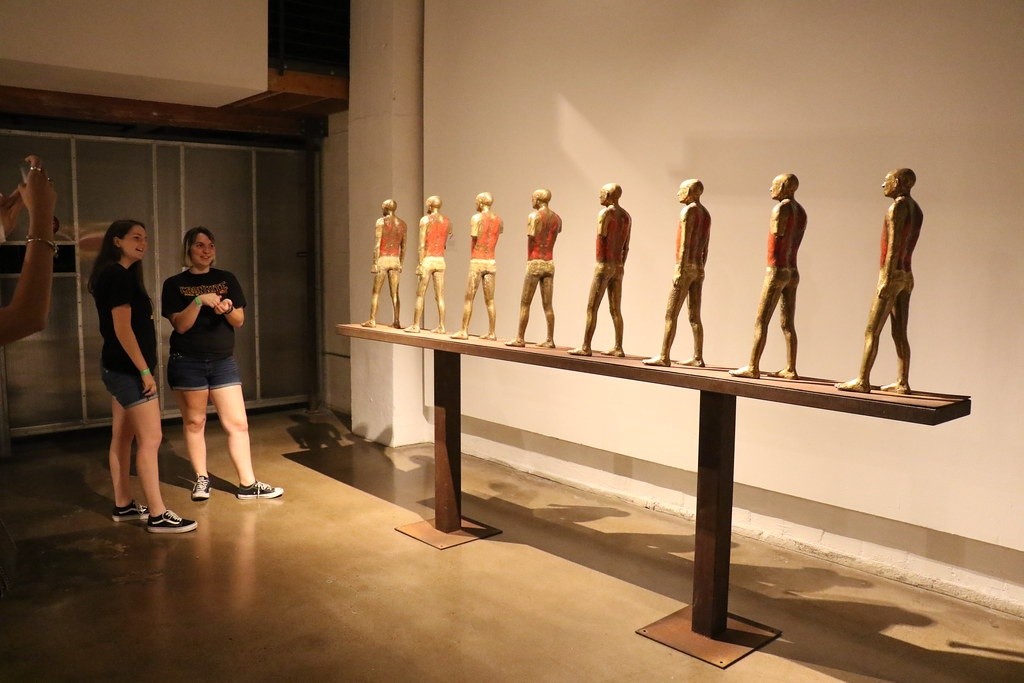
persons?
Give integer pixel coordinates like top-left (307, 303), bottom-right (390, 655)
top-left (1, 155), bottom-right (58, 345)
top-left (161, 226), bottom-right (284, 500)
top-left (88, 219), bottom-right (199, 535)
top-left (360, 168), bottom-right (926, 394)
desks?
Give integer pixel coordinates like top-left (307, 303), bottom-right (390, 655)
top-left (332, 324), bottom-right (972, 671)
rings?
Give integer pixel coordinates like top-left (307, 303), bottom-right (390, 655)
top-left (30, 166), bottom-right (43, 172)
top-left (47, 177), bottom-right (56, 183)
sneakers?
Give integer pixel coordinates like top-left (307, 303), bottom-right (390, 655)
top-left (111, 500), bottom-right (150, 521)
top-left (192, 473), bottom-right (210, 499)
top-left (147, 510), bottom-right (197, 533)
top-left (237, 479), bottom-right (283, 499)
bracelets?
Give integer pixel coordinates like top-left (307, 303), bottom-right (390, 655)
top-left (25, 237), bottom-right (59, 253)
top-left (225, 307), bottom-right (234, 314)
top-left (140, 368), bottom-right (150, 375)
top-left (194, 297), bottom-right (202, 306)
